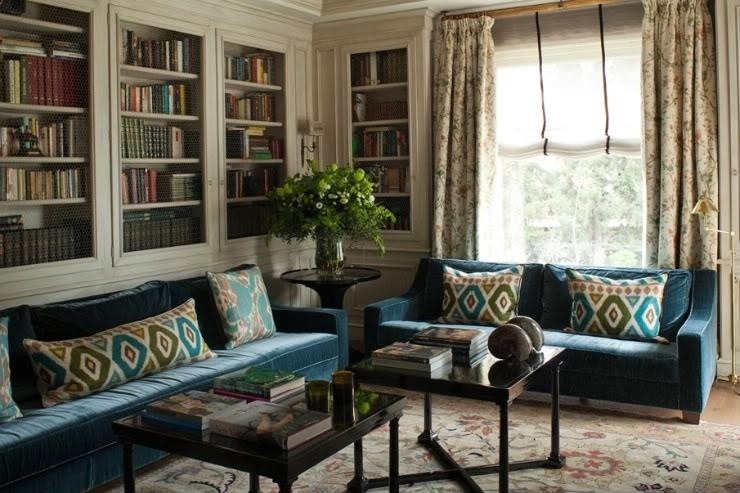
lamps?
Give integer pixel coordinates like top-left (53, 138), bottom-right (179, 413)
top-left (689, 195), bottom-right (735, 239)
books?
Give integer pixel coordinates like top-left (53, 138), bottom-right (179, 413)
top-left (228, 206), bottom-right (278, 240)
top-left (207, 400), bottom-right (335, 453)
top-left (411, 324), bottom-right (490, 368)
top-left (372, 337), bottom-right (453, 363)
top-left (341, 46), bottom-right (412, 234)
top-left (226, 168), bottom-right (277, 198)
top-left (119, 28), bottom-right (195, 73)
top-left (124, 217), bottom-right (201, 252)
top-left (121, 118), bottom-right (198, 159)
top-left (1, 167), bottom-right (90, 200)
top-left (226, 126), bottom-right (284, 159)
top-left (225, 90), bottom-right (275, 122)
top-left (226, 53), bottom-right (274, 85)
top-left (121, 82), bottom-right (191, 114)
top-left (1, 215), bottom-right (92, 267)
top-left (141, 361), bottom-right (310, 433)
top-left (368, 351), bottom-right (456, 372)
top-left (1, 38), bottom-right (87, 60)
top-left (1, 56), bottom-right (87, 107)
top-left (1, 115), bottom-right (89, 157)
top-left (122, 167), bottom-right (202, 203)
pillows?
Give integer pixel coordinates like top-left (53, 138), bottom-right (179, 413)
top-left (433, 258), bottom-right (668, 345)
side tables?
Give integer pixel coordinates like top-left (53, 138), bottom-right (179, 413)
top-left (277, 265), bottom-right (383, 364)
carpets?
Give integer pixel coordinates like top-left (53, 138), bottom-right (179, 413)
top-left (101, 362), bottom-right (738, 490)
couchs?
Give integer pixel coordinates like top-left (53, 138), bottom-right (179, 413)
top-left (364, 255), bottom-right (723, 427)
top-left (1, 262), bottom-right (352, 492)
